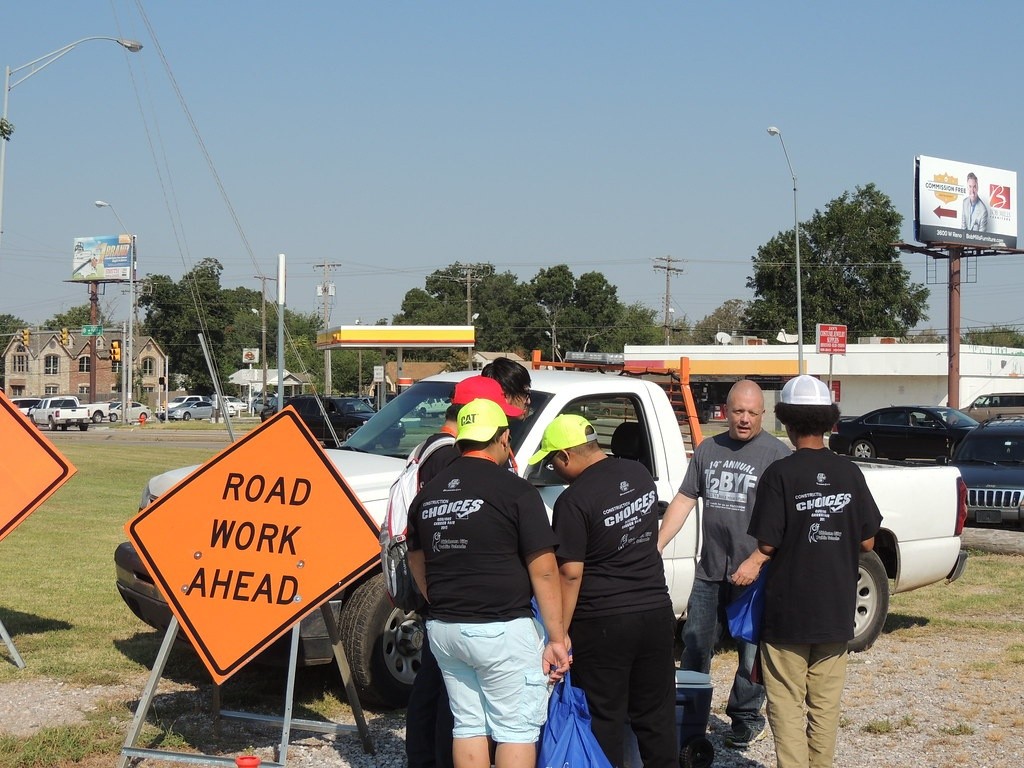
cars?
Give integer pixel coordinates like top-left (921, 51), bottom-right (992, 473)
top-left (935, 413), bottom-right (1024, 531)
top-left (110, 401), bottom-right (152, 423)
top-left (242, 389), bottom-right (292, 423)
top-left (827, 405), bottom-right (980, 461)
top-left (163, 401), bottom-right (213, 420)
top-left (411, 397), bottom-right (452, 418)
top-left (222, 395), bottom-right (248, 415)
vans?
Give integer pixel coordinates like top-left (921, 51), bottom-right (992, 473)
top-left (8, 398), bottom-right (41, 416)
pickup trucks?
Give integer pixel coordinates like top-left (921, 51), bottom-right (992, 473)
top-left (114, 367), bottom-right (969, 715)
top-left (29, 395), bottom-right (91, 431)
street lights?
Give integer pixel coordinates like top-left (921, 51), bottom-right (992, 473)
top-left (95, 200), bottom-right (134, 423)
top-left (766, 126), bottom-right (805, 376)
top-left (1, 37), bottom-right (145, 201)
top-left (251, 308), bottom-right (267, 405)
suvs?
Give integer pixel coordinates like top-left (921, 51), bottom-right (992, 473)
top-left (154, 395), bottom-right (212, 421)
top-left (283, 394), bottom-right (406, 452)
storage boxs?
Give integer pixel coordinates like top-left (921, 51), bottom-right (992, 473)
top-left (615, 669), bottom-right (714, 768)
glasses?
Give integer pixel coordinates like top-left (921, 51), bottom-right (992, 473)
top-left (506, 432), bottom-right (513, 444)
top-left (541, 450), bottom-right (561, 471)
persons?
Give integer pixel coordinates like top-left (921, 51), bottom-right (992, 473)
top-left (962, 172), bottom-right (988, 232)
top-left (404, 357), bottom-right (570, 768)
top-left (748, 373), bottom-right (883, 768)
top-left (528, 413), bottom-right (680, 768)
top-left (657, 381), bottom-right (795, 747)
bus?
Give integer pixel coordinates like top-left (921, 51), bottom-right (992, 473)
top-left (960, 392), bottom-right (1024, 422)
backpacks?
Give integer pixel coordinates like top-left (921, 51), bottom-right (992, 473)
top-left (379, 436), bottom-right (456, 611)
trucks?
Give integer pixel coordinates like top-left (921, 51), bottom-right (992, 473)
top-left (81, 403), bottom-right (110, 423)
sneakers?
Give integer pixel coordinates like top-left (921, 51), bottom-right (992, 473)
top-left (724, 718), bottom-right (767, 747)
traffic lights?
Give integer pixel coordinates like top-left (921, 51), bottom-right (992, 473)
top-left (108, 340), bottom-right (121, 363)
top-left (21, 329), bottom-right (29, 346)
top-left (60, 327), bottom-right (69, 347)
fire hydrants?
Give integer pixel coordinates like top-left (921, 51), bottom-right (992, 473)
top-left (138, 414), bottom-right (147, 426)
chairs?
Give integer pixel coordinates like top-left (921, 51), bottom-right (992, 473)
top-left (611, 421), bottom-right (642, 462)
top-left (911, 413), bottom-right (918, 425)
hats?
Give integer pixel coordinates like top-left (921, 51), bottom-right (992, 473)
top-left (528, 414), bottom-right (599, 466)
top-left (779, 375), bottom-right (832, 406)
top-left (452, 374), bottom-right (526, 416)
top-left (451, 397), bottom-right (508, 448)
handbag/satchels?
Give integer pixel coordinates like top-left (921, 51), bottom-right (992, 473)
top-left (726, 561), bottom-right (761, 647)
top-left (531, 596), bottom-right (614, 768)
